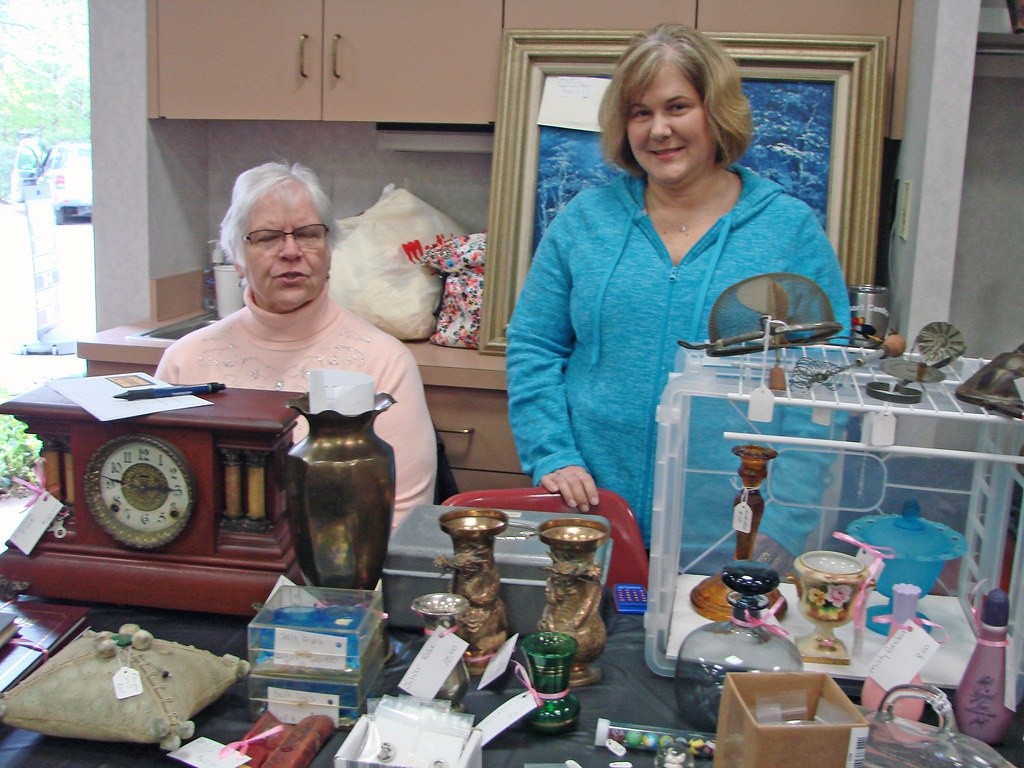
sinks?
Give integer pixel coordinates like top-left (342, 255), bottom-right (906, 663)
top-left (125, 308), bottom-right (222, 342)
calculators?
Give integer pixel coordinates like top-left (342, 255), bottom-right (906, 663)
top-left (614, 583), bottom-right (648, 614)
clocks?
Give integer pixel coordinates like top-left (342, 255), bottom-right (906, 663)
top-left (0, 378), bottom-right (309, 616)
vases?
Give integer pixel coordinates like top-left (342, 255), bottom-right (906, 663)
top-left (284, 389), bottom-right (398, 606)
top-left (410, 592), bottom-right (473, 707)
top-left (539, 518), bottom-right (611, 689)
top-left (522, 628), bottom-right (581, 736)
top-left (437, 507), bottom-right (511, 676)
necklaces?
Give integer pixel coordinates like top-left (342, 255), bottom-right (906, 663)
top-left (650, 171), bottom-right (729, 233)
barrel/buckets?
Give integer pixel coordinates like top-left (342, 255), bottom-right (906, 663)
top-left (848, 285), bottom-right (890, 348)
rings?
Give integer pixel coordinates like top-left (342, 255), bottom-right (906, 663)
top-left (776, 557), bottom-right (786, 566)
top-left (762, 552), bottom-right (774, 561)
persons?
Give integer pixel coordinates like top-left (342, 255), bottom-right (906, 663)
top-left (506, 23), bottom-right (852, 581)
top-left (152, 158), bottom-right (438, 541)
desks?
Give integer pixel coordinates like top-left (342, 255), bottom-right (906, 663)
top-left (0, 611), bottom-right (1024, 768)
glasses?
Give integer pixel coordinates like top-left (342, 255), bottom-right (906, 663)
top-left (243, 223), bottom-right (328, 251)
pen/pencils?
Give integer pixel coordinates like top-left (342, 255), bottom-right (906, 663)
top-left (111, 381), bottom-right (227, 401)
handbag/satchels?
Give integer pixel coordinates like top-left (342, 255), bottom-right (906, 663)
top-left (423, 232), bottom-right (487, 349)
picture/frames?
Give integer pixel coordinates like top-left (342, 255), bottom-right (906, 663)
top-left (477, 30), bottom-right (888, 355)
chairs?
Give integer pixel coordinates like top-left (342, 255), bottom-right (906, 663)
top-left (442, 487), bottom-right (649, 590)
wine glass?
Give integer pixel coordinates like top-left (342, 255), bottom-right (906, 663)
top-left (792, 551), bottom-right (869, 663)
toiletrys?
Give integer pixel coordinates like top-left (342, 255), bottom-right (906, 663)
top-left (203, 239), bottom-right (225, 310)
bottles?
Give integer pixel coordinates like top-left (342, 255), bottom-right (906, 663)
top-left (861, 582), bottom-right (926, 721)
top-left (410, 592), bottom-right (470, 707)
top-left (521, 630), bottom-right (579, 737)
top-left (951, 588), bottom-right (1015, 747)
top-left (674, 560), bottom-right (805, 734)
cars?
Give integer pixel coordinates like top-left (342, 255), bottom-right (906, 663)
top-left (11, 139), bottom-right (94, 226)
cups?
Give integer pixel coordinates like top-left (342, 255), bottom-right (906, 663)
top-left (213, 265), bottom-right (245, 319)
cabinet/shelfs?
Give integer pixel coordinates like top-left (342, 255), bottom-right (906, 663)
top-left (145, 0), bottom-right (504, 128)
top-left (499, 0), bottom-right (914, 140)
top-left (422, 384), bottom-right (532, 494)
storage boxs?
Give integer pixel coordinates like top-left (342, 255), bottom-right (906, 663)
top-left (382, 500), bottom-right (614, 641)
top-left (246, 585), bottom-right (384, 679)
top-left (711, 671), bottom-right (869, 768)
top-left (333, 711), bottom-right (485, 768)
top-left (635, 340), bottom-right (1024, 713)
top-left (245, 638), bottom-right (383, 728)
